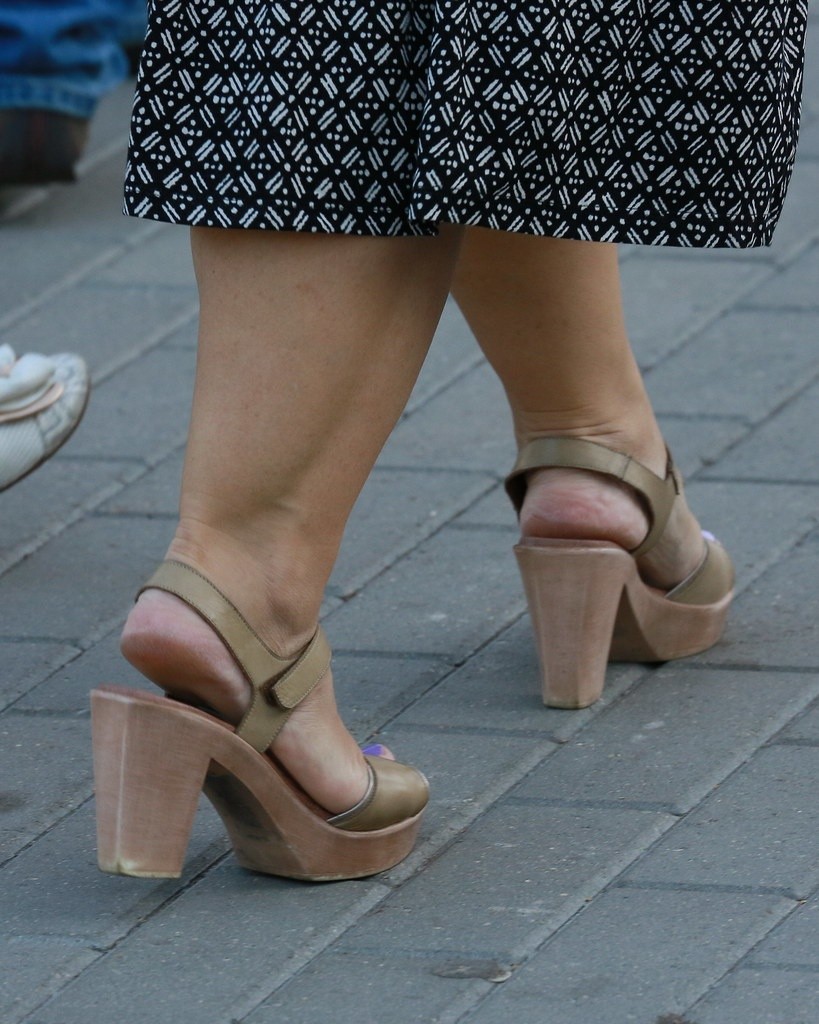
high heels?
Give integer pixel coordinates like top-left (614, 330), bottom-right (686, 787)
top-left (88, 562), bottom-right (431, 879)
top-left (504, 438), bottom-right (735, 709)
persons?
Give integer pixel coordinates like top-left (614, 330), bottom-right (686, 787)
top-left (88, 0), bottom-right (808, 882)
top-left (0, 0), bottom-right (148, 188)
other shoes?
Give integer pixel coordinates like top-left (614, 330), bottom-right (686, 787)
top-left (0, 343), bottom-right (91, 491)
top-left (1, 101), bottom-right (86, 202)
